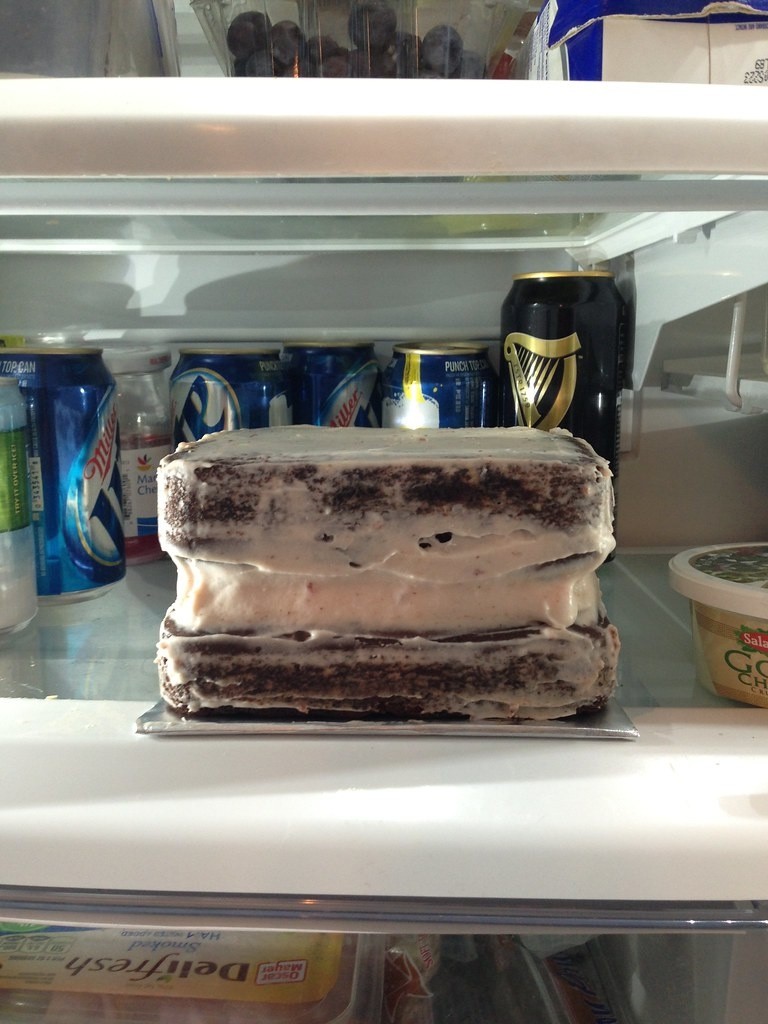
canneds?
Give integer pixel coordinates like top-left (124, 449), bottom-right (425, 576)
top-left (0, 273), bottom-right (632, 631)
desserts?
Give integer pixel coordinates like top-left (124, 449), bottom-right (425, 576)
top-left (156, 422), bottom-right (620, 723)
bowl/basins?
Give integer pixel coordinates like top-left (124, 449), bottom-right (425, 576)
top-left (191, 2), bottom-right (523, 79)
top-left (0, 905), bottom-right (388, 1022)
top-left (668, 537), bottom-right (768, 708)
